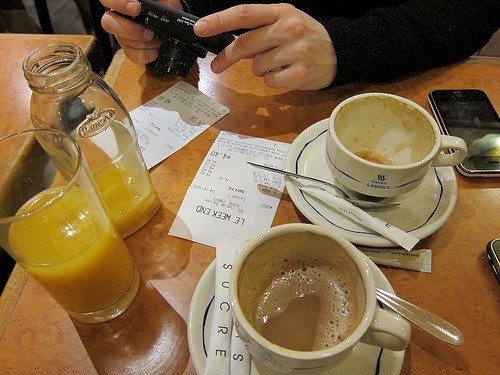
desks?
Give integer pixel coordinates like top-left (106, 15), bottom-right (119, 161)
top-left (0, 33), bottom-right (97, 219)
top-left (0, 49), bottom-right (500, 375)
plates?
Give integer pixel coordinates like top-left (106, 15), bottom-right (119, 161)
top-left (283, 116), bottom-right (458, 248)
top-left (187, 258), bottom-right (406, 375)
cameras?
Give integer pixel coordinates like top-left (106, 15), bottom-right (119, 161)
top-left (115, 0), bottom-right (235, 78)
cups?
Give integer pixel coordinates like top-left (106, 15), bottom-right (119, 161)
top-left (219, 214), bottom-right (411, 375)
top-left (0, 128), bottom-right (140, 325)
top-left (324, 91), bottom-right (468, 200)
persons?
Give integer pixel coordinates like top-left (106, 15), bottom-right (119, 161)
top-left (100, 0), bottom-right (500, 92)
top-left (0, 0), bottom-right (121, 77)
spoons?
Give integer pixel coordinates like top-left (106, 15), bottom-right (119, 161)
top-left (248, 162), bottom-right (400, 210)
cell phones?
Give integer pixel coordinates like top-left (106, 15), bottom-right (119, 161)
top-left (428, 89), bottom-right (500, 178)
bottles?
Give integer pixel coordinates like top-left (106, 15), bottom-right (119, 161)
top-left (22, 39), bottom-right (160, 239)
